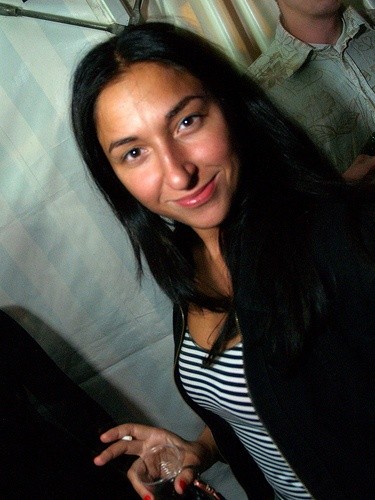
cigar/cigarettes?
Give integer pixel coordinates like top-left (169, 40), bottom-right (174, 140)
top-left (121, 435), bottom-right (132, 441)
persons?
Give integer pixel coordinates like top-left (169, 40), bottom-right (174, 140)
top-left (0, 0), bottom-right (375, 500)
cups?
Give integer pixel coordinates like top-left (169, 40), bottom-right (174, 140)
top-left (134, 444), bottom-right (198, 500)
